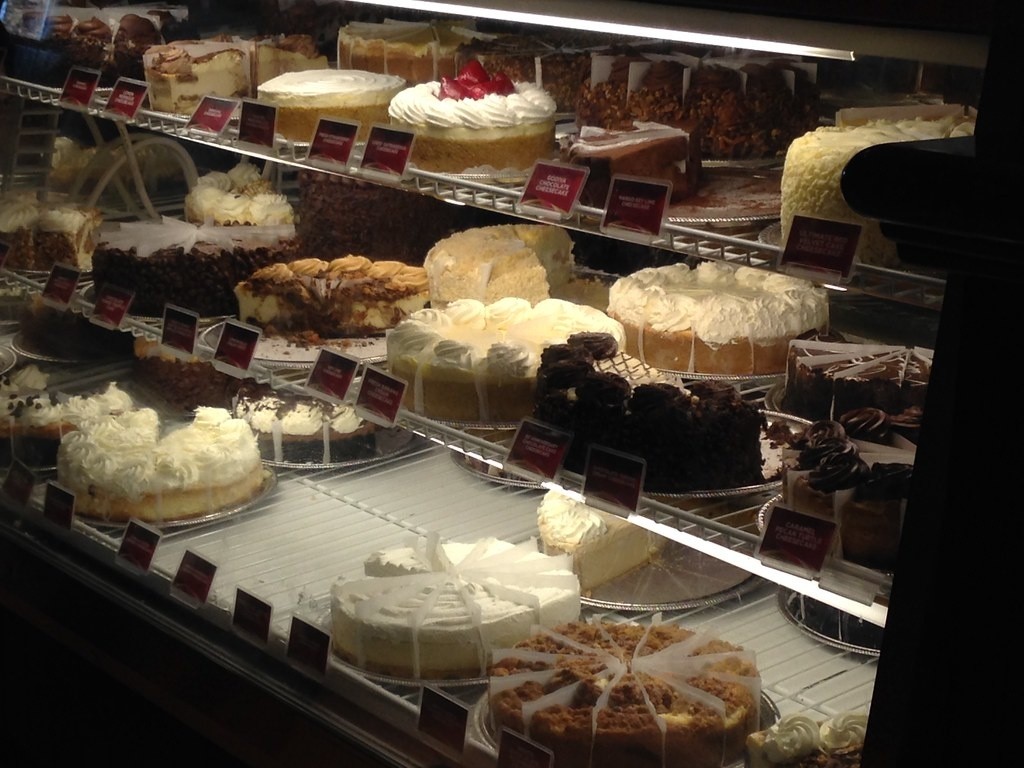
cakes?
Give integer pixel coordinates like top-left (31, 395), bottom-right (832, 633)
top-left (0, 0), bottom-right (979, 768)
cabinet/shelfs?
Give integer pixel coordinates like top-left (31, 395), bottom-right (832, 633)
top-left (0, 0), bottom-right (1024, 768)
